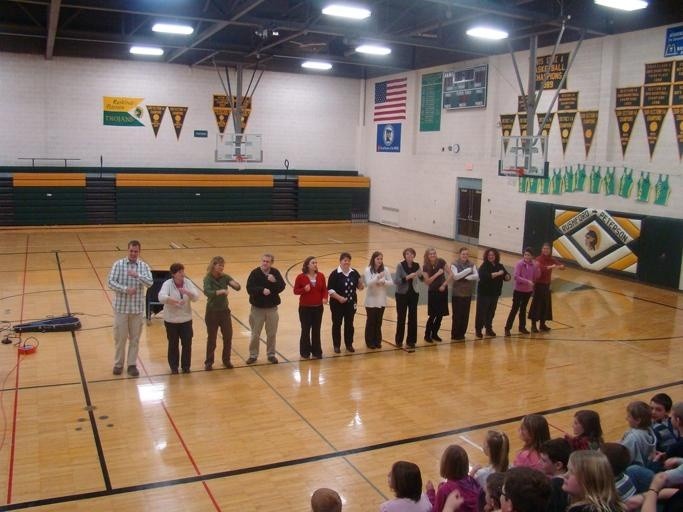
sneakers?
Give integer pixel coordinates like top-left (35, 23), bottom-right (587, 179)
top-left (112, 367), bottom-right (139, 377)
top-left (474, 329), bottom-right (497, 337)
top-left (204, 360), bottom-right (233, 370)
top-left (246, 356), bottom-right (278, 365)
top-left (450, 335), bottom-right (465, 340)
top-left (298, 352), bottom-right (321, 361)
top-left (171, 367), bottom-right (190, 374)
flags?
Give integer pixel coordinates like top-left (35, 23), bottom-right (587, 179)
top-left (374, 78), bottom-right (407, 121)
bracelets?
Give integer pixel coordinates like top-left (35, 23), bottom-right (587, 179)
top-left (442, 284), bottom-right (447, 287)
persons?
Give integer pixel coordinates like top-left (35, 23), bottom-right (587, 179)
top-left (449, 248), bottom-right (478, 341)
top-left (505, 248), bottom-right (542, 337)
top-left (421, 248), bottom-right (454, 342)
top-left (528, 242), bottom-right (565, 332)
top-left (157, 264), bottom-right (199, 374)
top-left (204, 257), bottom-right (241, 369)
top-left (328, 252), bottom-right (364, 354)
top-left (293, 256), bottom-right (329, 360)
top-left (246, 254), bottom-right (285, 365)
top-left (585, 228), bottom-right (599, 251)
top-left (394, 249), bottom-right (426, 347)
top-left (108, 241), bottom-right (154, 377)
top-left (476, 248), bottom-right (511, 337)
top-left (361, 252), bottom-right (393, 349)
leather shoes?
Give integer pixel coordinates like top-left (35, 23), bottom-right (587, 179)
top-left (423, 334), bottom-right (441, 344)
top-left (395, 341), bottom-right (416, 349)
top-left (333, 345), bottom-right (354, 353)
top-left (531, 325), bottom-right (551, 333)
top-left (367, 344), bottom-right (381, 349)
top-left (503, 327), bottom-right (529, 336)
